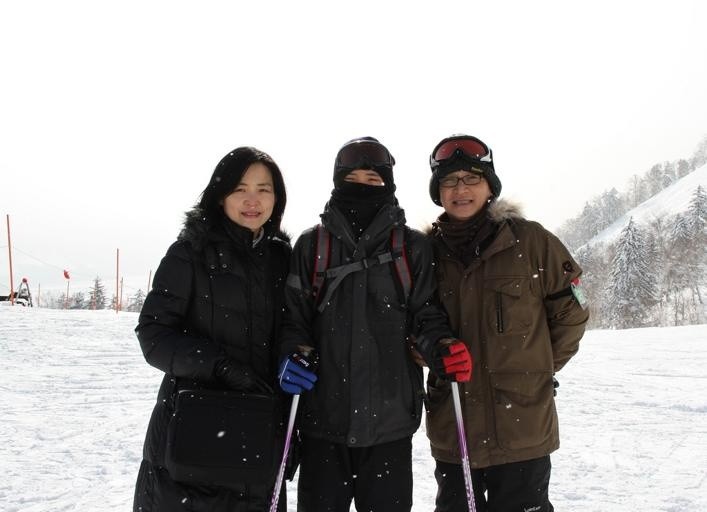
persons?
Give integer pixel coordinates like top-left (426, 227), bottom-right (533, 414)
top-left (131, 145), bottom-right (323, 512)
top-left (408, 134), bottom-right (593, 512)
top-left (281, 135), bottom-right (474, 512)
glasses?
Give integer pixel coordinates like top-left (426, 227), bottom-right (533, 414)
top-left (437, 174), bottom-right (485, 187)
top-left (430, 137), bottom-right (493, 166)
top-left (338, 141), bottom-right (394, 167)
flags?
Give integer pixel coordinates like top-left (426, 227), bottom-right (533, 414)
top-left (63, 269), bottom-right (70, 280)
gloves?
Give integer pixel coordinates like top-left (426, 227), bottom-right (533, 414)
top-left (279, 353), bottom-right (317, 394)
top-left (434, 337), bottom-right (472, 383)
top-left (245, 344), bottom-right (277, 378)
top-left (216, 356), bottom-right (275, 397)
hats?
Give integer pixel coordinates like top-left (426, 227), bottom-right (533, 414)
top-left (333, 137), bottom-right (395, 198)
top-left (428, 133), bottom-right (501, 207)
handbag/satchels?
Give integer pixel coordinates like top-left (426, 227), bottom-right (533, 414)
top-left (166, 386), bottom-right (285, 499)
